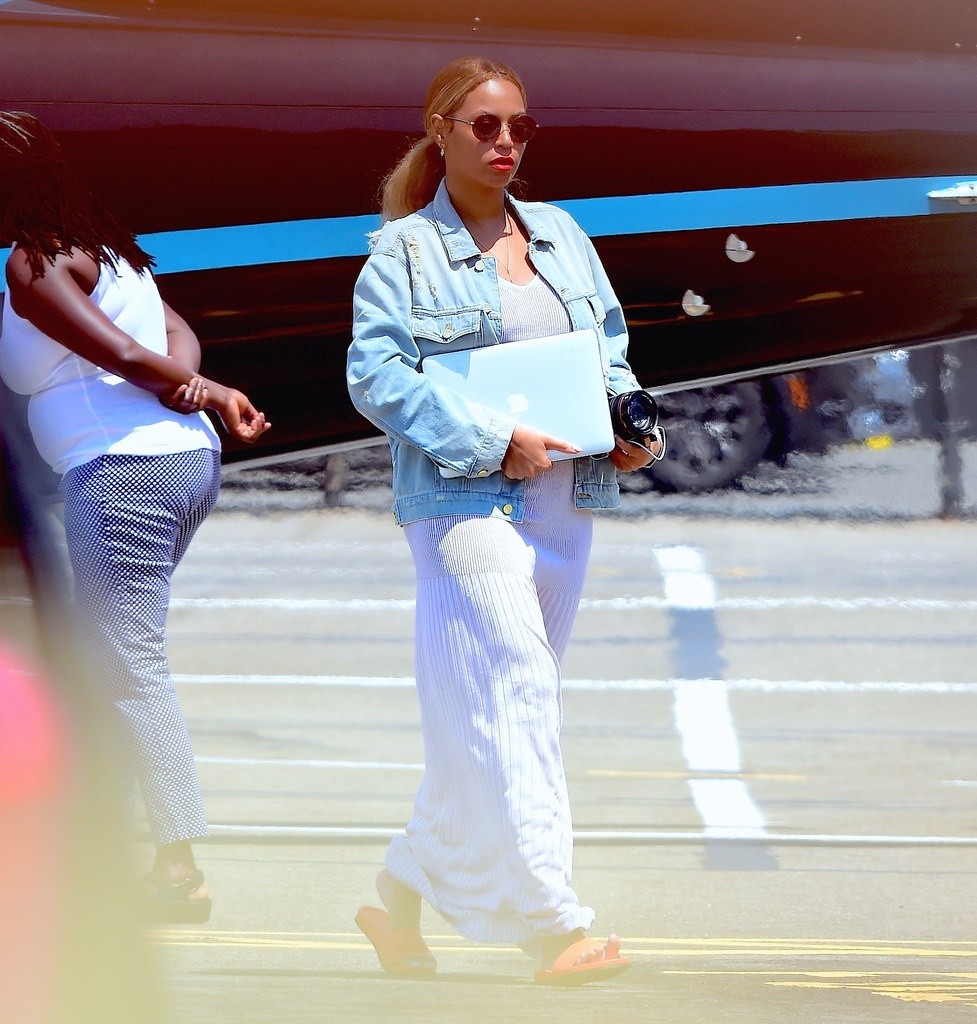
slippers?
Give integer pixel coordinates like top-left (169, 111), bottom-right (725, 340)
top-left (355, 904), bottom-right (437, 979)
top-left (532, 936), bottom-right (631, 986)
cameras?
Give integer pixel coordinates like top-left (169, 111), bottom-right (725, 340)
top-left (608, 390), bottom-right (659, 447)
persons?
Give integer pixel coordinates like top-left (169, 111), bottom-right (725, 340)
top-left (347, 55), bottom-right (667, 988)
top-left (0, 108), bottom-right (272, 922)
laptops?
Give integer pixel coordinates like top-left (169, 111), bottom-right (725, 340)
top-left (424, 330), bottom-right (614, 480)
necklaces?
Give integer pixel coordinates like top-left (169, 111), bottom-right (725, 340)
top-left (467, 206), bottom-right (514, 284)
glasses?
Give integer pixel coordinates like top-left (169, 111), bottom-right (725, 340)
top-left (445, 107), bottom-right (540, 147)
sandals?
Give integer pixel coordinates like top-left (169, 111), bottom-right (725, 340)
top-left (144, 868), bottom-right (212, 927)
